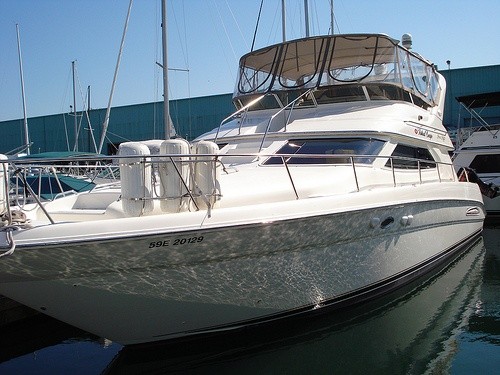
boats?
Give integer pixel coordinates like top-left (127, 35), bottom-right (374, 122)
top-left (101, 236), bottom-right (489, 375)
top-left (0, 0), bottom-right (499, 346)
